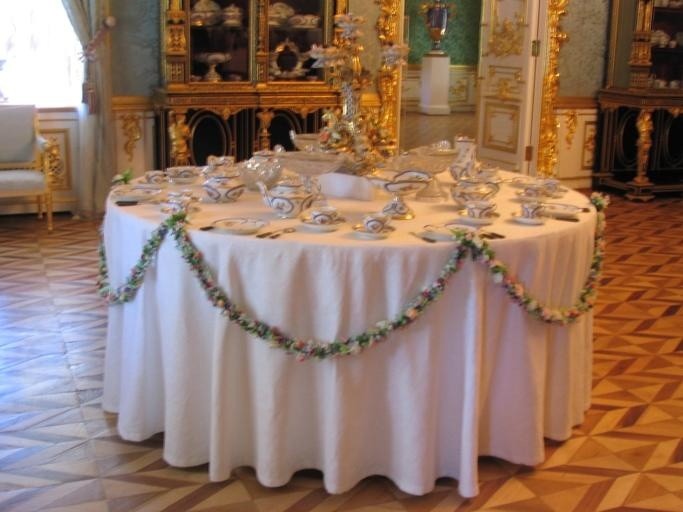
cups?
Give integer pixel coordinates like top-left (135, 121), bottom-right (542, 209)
top-left (208, 155), bottom-right (236, 173)
top-left (651, 0), bottom-right (682, 92)
top-left (144, 171), bottom-right (166, 184)
top-left (476, 162), bottom-right (500, 177)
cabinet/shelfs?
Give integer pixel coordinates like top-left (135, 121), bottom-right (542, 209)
top-left (652, 0), bottom-right (682, 93)
top-left (591, 86), bottom-right (682, 201)
top-left (149, 83), bottom-right (344, 173)
top-left (157, 0), bottom-right (347, 88)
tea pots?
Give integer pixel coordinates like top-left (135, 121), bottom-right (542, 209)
top-left (242, 145), bottom-right (288, 193)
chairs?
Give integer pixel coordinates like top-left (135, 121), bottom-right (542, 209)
top-left (0, 104), bottom-right (59, 233)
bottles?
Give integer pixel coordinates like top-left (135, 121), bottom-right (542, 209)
top-left (450, 136), bottom-right (478, 181)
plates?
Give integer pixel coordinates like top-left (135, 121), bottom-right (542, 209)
top-left (193, 0), bottom-right (222, 26)
top-left (113, 173), bottom-right (580, 242)
top-left (267, 2), bottom-right (295, 27)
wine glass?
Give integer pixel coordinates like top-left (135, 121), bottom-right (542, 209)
top-left (278, 128), bottom-right (457, 202)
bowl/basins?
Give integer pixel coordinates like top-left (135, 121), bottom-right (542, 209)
top-left (288, 14), bottom-right (321, 29)
top-left (167, 165), bottom-right (196, 184)
